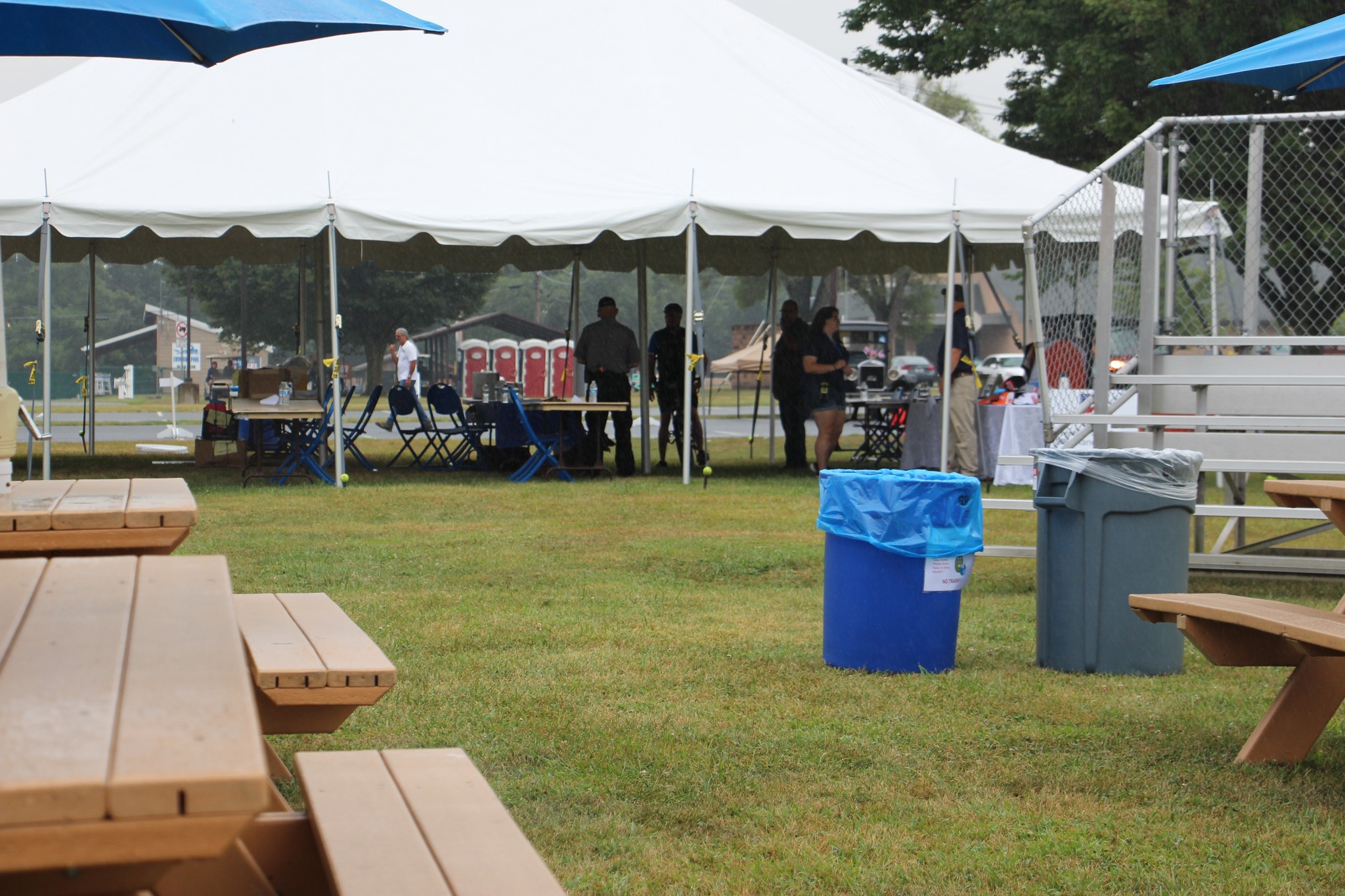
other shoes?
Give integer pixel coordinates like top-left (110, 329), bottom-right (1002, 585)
top-left (415, 421), bottom-right (433, 432)
top-left (375, 422), bottom-right (393, 432)
top-left (810, 462), bottom-right (831, 478)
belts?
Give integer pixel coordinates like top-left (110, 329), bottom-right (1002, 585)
top-left (951, 371), bottom-right (973, 379)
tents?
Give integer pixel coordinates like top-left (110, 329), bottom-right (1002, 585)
top-left (0, 0), bottom-right (1235, 486)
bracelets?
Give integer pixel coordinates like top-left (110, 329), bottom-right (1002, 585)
top-left (833, 364), bottom-right (836, 371)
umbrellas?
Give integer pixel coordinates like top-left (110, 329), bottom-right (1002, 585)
top-left (1, 0), bottom-right (447, 68)
top-left (1148, 12), bottom-right (1344, 92)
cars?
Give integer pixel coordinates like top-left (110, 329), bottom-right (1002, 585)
top-left (891, 356), bottom-right (936, 390)
top-left (976, 353), bottom-right (1029, 384)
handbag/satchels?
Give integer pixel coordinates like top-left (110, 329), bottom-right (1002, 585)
top-left (1003, 376), bottom-right (1027, 398)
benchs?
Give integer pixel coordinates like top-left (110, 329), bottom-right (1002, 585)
top-left (232, 591), bottom-right (399, 734)
top-left (1128, 592), bottom-right (1345, 765)
top-left (293, 747), bottom-right (567, 895)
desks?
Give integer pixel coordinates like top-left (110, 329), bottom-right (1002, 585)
top-left (0, 556), bottom-right (357, 896)
top-left (899, 399), bottom-right (1043, 493)
top-left (517, 399), bottom-right (629, 485)
top-left (1, 479), bottom-right (198, 558)
top-left (1232, 478), bottom-right (1344, 767)
top-left (843, 392), bottom-right (943, 470)
top-left (232, 395), bottom-right (329, 490)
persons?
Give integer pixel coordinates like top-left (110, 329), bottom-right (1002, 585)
top-left (206, 362), bottom-right (221, 385)
top-left (802, 306), bottom-right (852, 479)
top-left (573, 296), bottom-right (641, 475)
top-left (772, 300), bottom-right (811, 469)
top-left (936, 284), bottom-right (980, 478)
top-left (223, 360), bottom-right (236, 379)
top-left (649, 303), bottom-right (709, 466)
top-left (375, 327), bottom-right (433, 434)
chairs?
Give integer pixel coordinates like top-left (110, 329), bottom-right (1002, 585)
top-left (847, 387), bottom-right (921, 467)
top-left (268, 383), bottom-right (575, 488)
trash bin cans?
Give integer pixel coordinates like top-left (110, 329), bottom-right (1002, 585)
top-left (496, 403), bottom-right (522, 447)
top-left (819, 470), bottom-right (980, 674)
top-left (1028, 449), bottom-right (1202, 677)
top-left (238, 419), bottom-right (280, 449)
top-left (472, 372), bottom-right (499, 399)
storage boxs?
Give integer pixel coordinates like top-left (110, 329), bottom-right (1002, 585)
top-left (246, 366), bottom-right (311, 399)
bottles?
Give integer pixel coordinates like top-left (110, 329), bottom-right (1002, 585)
top-left (279, 381), bottom-right (290, 406)
top-left (502, 384), bottom-right (509, 403)
top-left (494, 382), bottom-right (502, 401)
top-left (482, 382), bottom-right (489, 403)
top-left (1058, 373), bottom-right (1070, 389)
top-left (513, 384), bottom-right (519, 397)
top-left (588, 381), bottom-right (598, 403)
top-left (860, 388), bottom-right (868, 401)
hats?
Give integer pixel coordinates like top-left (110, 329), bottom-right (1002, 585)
top-left (941, 284), bottom-right (964, 302)
top-left (597, 296), bottom-right (616, 317)
top-left (990, 392), bottom-right (1014, 406)
top-left (1012, 392), bottom-right (1038, 405)
